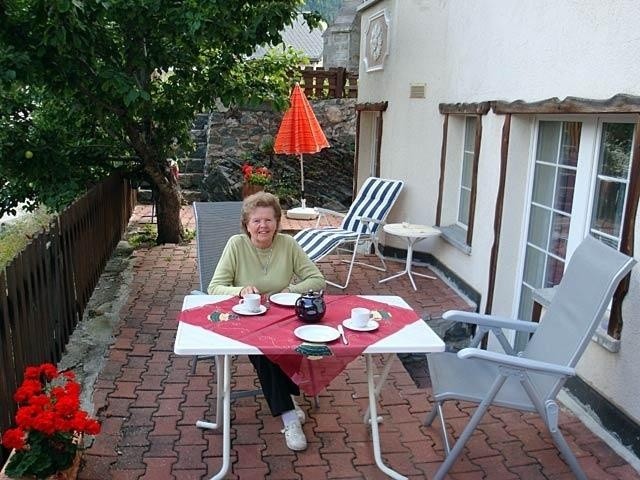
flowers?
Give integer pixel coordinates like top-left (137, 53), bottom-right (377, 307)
top-left (0, 362), bottom-right (103, 480)
top-left (242, 163), bottom-right (273, 186)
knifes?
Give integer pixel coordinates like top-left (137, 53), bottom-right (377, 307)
top-left (338, 324), bottom-right (348, 345)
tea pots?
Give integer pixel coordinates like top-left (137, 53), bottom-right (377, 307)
top-left (294, 288), bottom-right (326, 323)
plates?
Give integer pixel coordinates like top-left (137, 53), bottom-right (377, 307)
top-left (269, 293), bottom-right (302, 306)
top-left (293, 324), bottom-right (341, 343)
top-left (232, 304), bottom-right (267, 316)
top-left (342, 318), bottom-right (380, 332)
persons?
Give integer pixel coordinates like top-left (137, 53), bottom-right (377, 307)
top-left (207, 190), bottom-right (328, 453)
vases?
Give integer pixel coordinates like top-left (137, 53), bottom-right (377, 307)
top-left (241, 183), bottom-right (265, 199)
top-left (0, 431), bottom-right (83, 480)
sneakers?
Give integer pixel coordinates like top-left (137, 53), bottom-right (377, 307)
top-left (282, 399), bottom-right (308, 452)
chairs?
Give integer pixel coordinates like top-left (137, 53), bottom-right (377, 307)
top-left (192, 201), bottom-right (321, 400)
top-left (424, 234), bottom-right (638, 480)
top-left (293, 177), bottom-right (404, 289)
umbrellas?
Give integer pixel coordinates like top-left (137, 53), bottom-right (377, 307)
top-left (274, 81), bottom-right (332, 209)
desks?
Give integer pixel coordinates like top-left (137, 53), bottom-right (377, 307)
top-left (174, 292), bottom-right (446, 480)
top-left (379, 223), bottom-right (442, 292)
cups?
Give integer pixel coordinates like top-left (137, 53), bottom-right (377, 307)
top-left (350, 307), bottom-right (375, 328)
top-left (239, 293), bottom-right (261, 312)
top-left (401, 221), bottom-right (409, 229)
